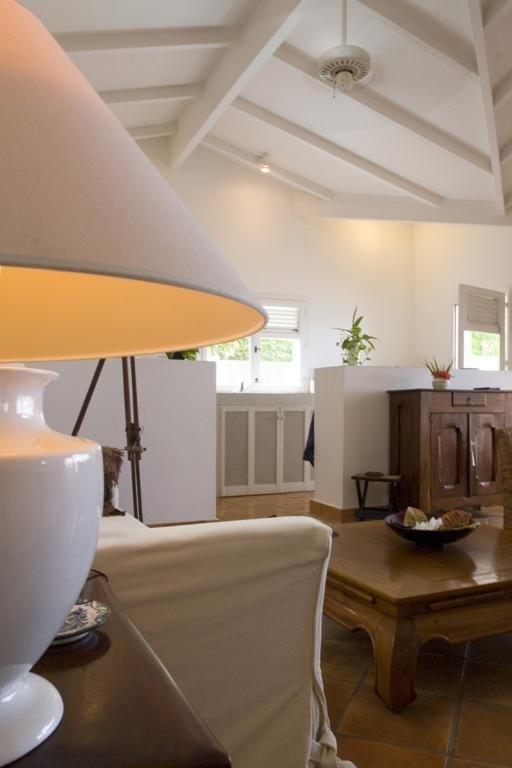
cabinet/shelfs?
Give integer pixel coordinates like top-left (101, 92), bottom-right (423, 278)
top-left (386, 389), bottom-right (512, 513)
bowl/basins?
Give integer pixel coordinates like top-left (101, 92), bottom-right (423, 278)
top-left (384, 505), bottom-right (482, 550)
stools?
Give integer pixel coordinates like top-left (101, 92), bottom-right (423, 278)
top-left (351, 472), bottom-right (400, 521)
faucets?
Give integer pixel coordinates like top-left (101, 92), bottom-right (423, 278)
top-left (240, 377), bottom-right (259, 393)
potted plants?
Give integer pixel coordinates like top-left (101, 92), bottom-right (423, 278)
top-left (332, 306), bottom-right (377, 365)
top-left (422, 355), bottom-right (455, 388)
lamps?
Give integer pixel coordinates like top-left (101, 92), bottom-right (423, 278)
top-left (319, 0), bottom-right (371, 92)
top-left (1, 0), bottom-right (268, 759)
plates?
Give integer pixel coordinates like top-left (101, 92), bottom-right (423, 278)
top-left (52, 597), bottom-right (114, 645)
